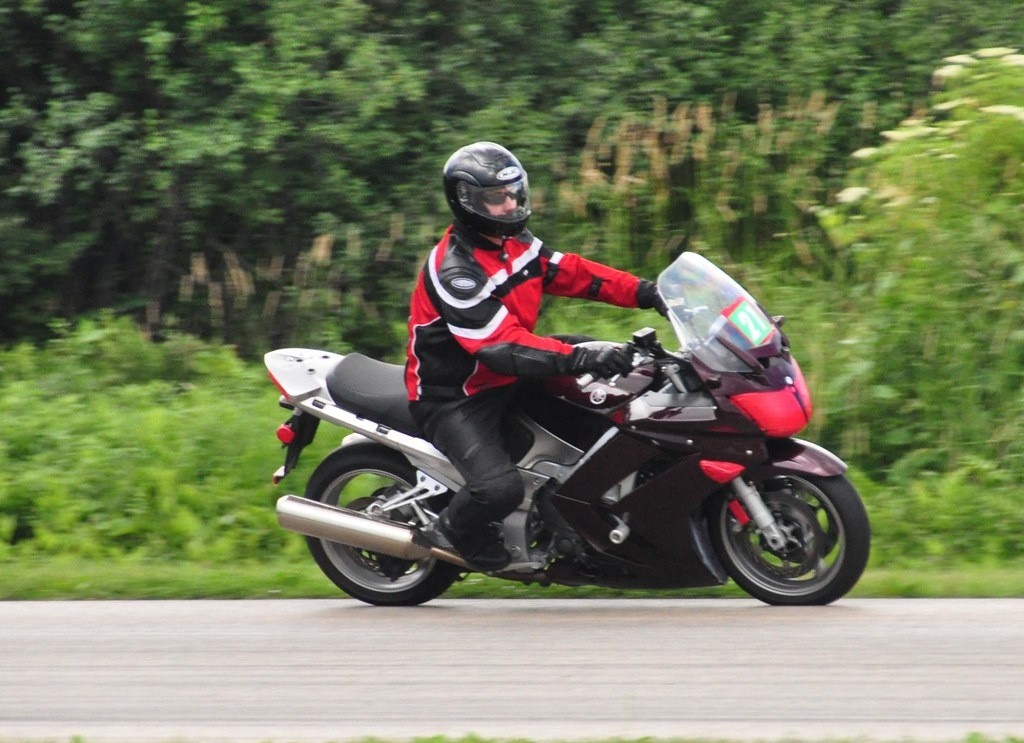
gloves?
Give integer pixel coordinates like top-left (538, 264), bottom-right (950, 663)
top-left (567, 341), bottom-right (634, 381)
top-left (637, 278), bottom-right (687, 321)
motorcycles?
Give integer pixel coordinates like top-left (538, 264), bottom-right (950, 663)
top-left (262, 251), bottom-right (872, 607)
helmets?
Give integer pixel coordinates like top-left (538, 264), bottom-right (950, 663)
top-left (442, 141), bottom-right (531, 239)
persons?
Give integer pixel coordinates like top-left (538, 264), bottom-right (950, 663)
top-left (404, 142), bottom-right (693, 574)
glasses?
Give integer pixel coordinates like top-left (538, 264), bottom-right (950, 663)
top-left (481, 186), bottom-right (520, 205)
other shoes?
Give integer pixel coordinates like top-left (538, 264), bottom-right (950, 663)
top-left (434, 507), bottom-right (511, 572)
